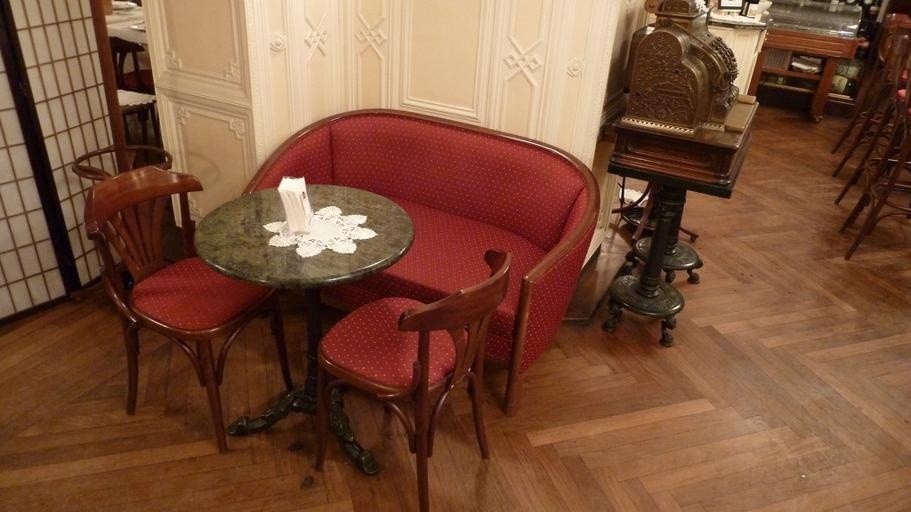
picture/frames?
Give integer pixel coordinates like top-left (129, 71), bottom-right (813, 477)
top-left (718, 0), bottom-right (743, 9)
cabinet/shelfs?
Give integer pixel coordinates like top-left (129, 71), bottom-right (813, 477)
top-left (747, 37), bottom-right (862, 124)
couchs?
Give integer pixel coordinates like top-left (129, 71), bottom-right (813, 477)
top-left (243, 109), bottom-right (600, 414)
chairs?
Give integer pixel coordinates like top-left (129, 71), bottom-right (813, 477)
top-left (830, 14), bottom-right (910, 260)
top-left (84, 166), bottom-right (292, 453)
top-left (117, 89), bottom-right (161, 158)
top-left (302, 247), bottom-right (514, 512)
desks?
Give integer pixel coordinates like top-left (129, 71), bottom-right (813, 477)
top-left (106, 9), bottom-right (147, 95)
top-left (195, 184), bottom-right (415, 478)
